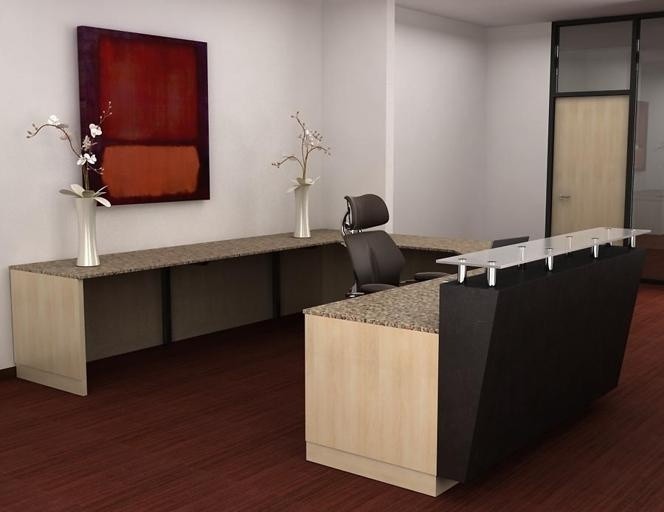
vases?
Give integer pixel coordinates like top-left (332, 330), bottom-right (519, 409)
top-left (289, 183), bottom-right (312, 238)
top-left (70, 196), bottom-right (100, 267)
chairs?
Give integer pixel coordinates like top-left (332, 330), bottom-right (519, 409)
top-left (341, 194), bottom-right (453, 300)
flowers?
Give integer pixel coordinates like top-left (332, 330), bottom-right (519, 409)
top-left (270, 110), bottom-right (333, 197)
top-left (23, 100), bottom-right (115, 210)
